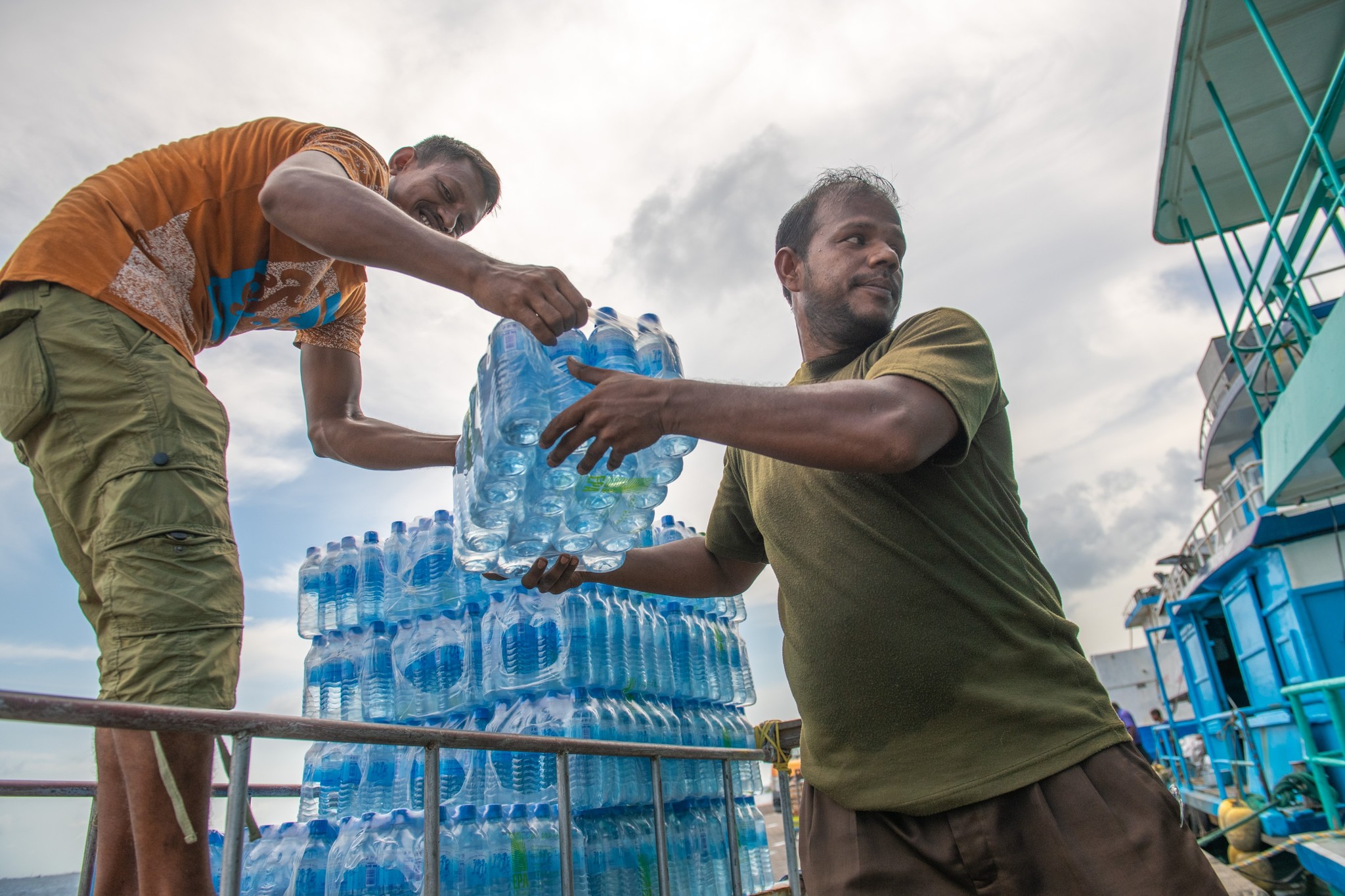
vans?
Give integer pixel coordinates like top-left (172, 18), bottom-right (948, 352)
top-left (771, 745), bottom-right (803, 811)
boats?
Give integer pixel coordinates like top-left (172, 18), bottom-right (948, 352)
top-left (1138, 0), bottom-right (1344, 893)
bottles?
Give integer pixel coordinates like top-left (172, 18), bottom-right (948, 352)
top-left (527, 469), bottom-right (570, 519)
top-left (209, 797), bottom-right (774, 896)
top-left (633, 313), bottom-right (699, 458)
top-left (574, 478), bottom-right (619, 510)
top-left (498, 548), bottom-right (538, 579)
top-left (621, 477), bottom-right (667, 509)
top-left (297, 688), bottom-right (764, 822)
top-left (488, 317), bottom-right (552, 446)
top-left (635, 448), bottom-right (683, 484)
top-left (533, 449), bottom-right (581, 492)
top-left (559, 581), bottom-right (758, 707)
top-left (507, 529), bottom-right (552, 558)
top-left (452, 467), bottom-right (498, 573)
top-left (639, 515), bottom-right (748, 622)
top-left (584, 453), bottom-right (637, 489)
top-left (477, 353), bottom-right (536, 478)
top-left (298, 509), bottom-right (490, 639)
top-left (454, 433), bottom-right (509, 552)
top-left (463, 410), bottom-right (516, 529)
top-left (543, 328), bottom-right (596, 455)
top-left (302, 586), bottom-right (558, 723)
top-left (514, 497), bottom-right (561, 538)
top-left (543, 509), bottom-right (654, 574)
top-left (589, 307), bottom-right (644, 451)
top-left (468, 383), bottom-right (524, 506)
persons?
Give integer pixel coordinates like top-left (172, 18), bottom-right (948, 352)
top-left (1111, 702), bottom-right (1155, 765)
top-left (1149, 709), bottom-right (1189, 783)
top-left (483, 163), bottom-right (1235, 896)
top-left (0, 114), bottom-right (595, 895)
top-left (1176, 732), bottom-right (1206, 776)
